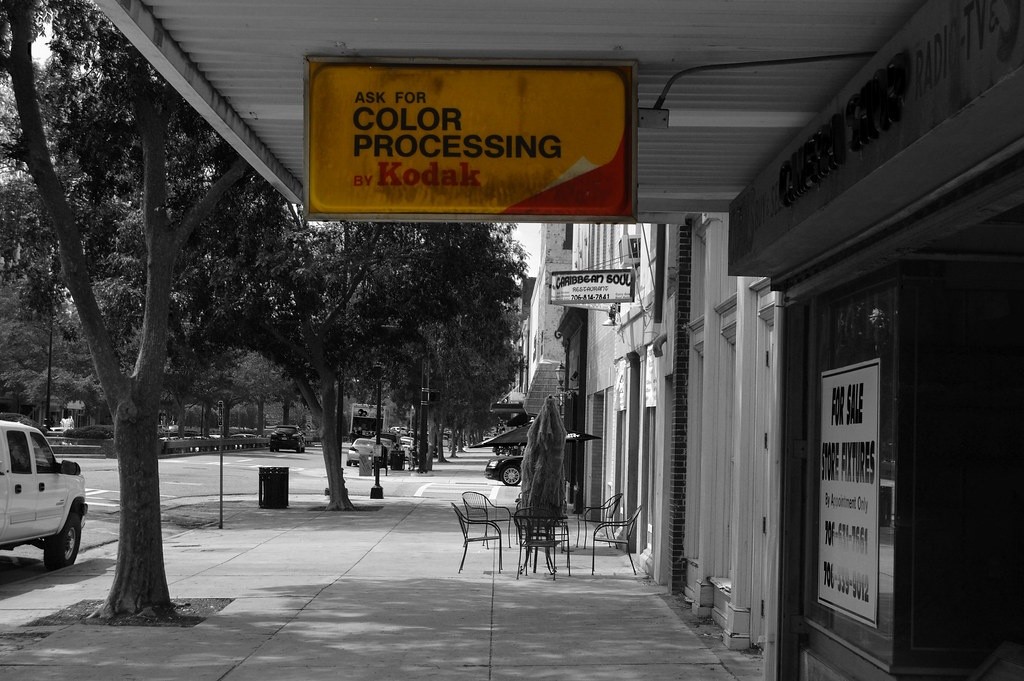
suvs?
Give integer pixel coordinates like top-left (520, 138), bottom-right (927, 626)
top-left (270, 424), bottom-right (305, 453)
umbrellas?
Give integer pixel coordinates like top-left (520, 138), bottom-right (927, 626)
top-left (470, 417), bottom-right (602, 448)
top-left (520, 394), bottom-right (566, 572)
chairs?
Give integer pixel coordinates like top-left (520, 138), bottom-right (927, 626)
top-left (461, 491), bottom-right (512, 550)
top-left (450, 501), bottom-right (504, 575)
top-left (590, 505), bottom-right (643, 575)
top-left (575, 493), bottom-right (623, 550)
top-left (513, 491), bottom-right (572, 581)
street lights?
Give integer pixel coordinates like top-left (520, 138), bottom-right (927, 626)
top-left (368, 364), bottom-right (385, 499)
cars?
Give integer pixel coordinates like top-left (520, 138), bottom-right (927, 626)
top-left (401, 430), bottom-right (453, 460)
top-left (346, 438), bottom-right (386, 466)
top-left (0, 419), bottom-right (88, 571)
top-left (485, 455), bottom-right (523, 486)
top-left (371, 437), bottom-right (394, 468)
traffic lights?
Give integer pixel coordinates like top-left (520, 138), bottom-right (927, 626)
top-left (218, 400), bottom-right (223, 426)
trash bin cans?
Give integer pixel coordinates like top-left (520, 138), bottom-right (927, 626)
top-left (258, 467), bottom-right (289, 509)
top-left (392, 451), bottom-right (406, 471)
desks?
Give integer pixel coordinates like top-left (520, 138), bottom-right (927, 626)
top-left (511, 514), bottom-right (572, 577)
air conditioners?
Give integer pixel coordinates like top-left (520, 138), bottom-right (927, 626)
top-left (617, 234), bottom-right (640, 269)
